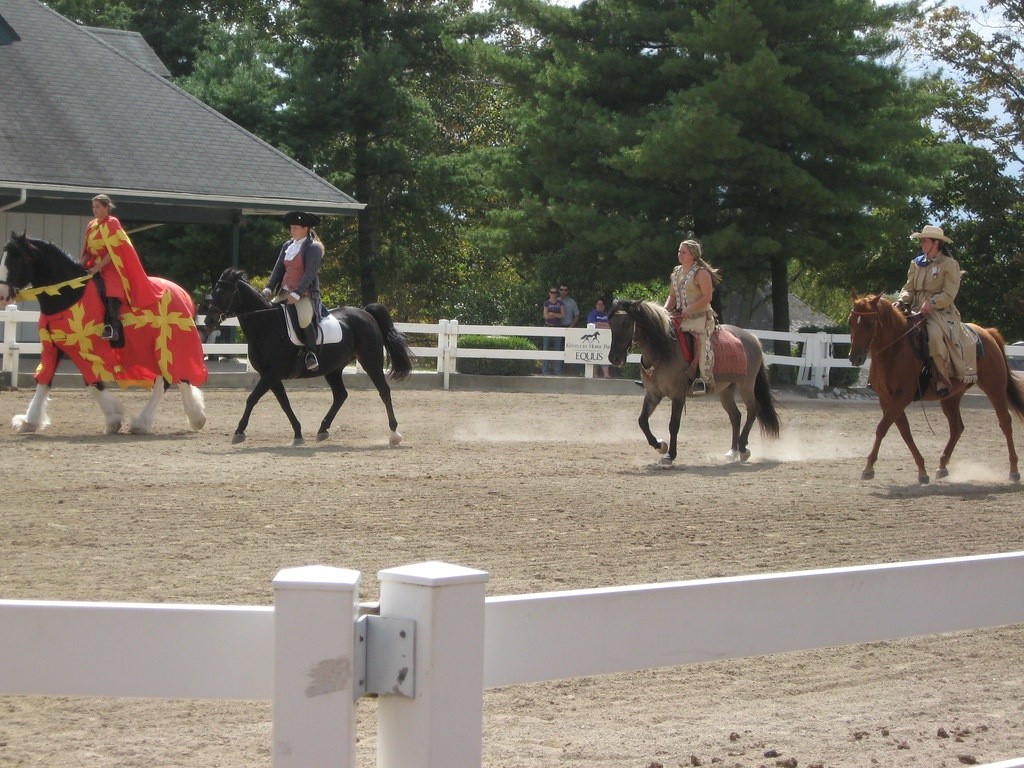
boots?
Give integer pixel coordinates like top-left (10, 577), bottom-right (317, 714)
top-left (299, 323), bottom-right (318, 370)
top-left (102, 296), bottom-right (121, 341)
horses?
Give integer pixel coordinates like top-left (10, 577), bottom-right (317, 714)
top-left (0, 228), bottom-right (208, 434)
top-left (608, 297), bottom-right (781, 466)
top-left (848, 285), bottom-right (1024, 485)
top-left (204, 266), bottom-right (414, 448)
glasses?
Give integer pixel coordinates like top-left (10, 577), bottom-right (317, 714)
top-left (560, 288), bottom-right (568, 291)
top-left (550, 291), bottom-right (557, 294)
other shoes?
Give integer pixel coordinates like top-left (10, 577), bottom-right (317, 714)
top-left (936, 387), bottom-right (948, 398)
top-left (693, 382), bottom-right (708, 395)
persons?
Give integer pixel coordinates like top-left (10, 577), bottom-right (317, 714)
top-left (195, 291), bottom-right (228, 363)
top-left (891, 222), bottom-right (978, 398)
top-left (548, 281), bottom-right (584, 377)
top-left (634, 239), bottom-right (723, 394)
top-left (261, 210), bottom-right (328, 374)
top-left (586, 296), bottom-right (612, 379)
top-left (542, 285), bottom-right (566, 376)
top-left (80, 194), bottom-right (162, 338)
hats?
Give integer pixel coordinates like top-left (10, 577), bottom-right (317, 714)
top-left (283, 212), bottom-right (320, 229)
top-left (910, 225), bottom-right (953, 243)
